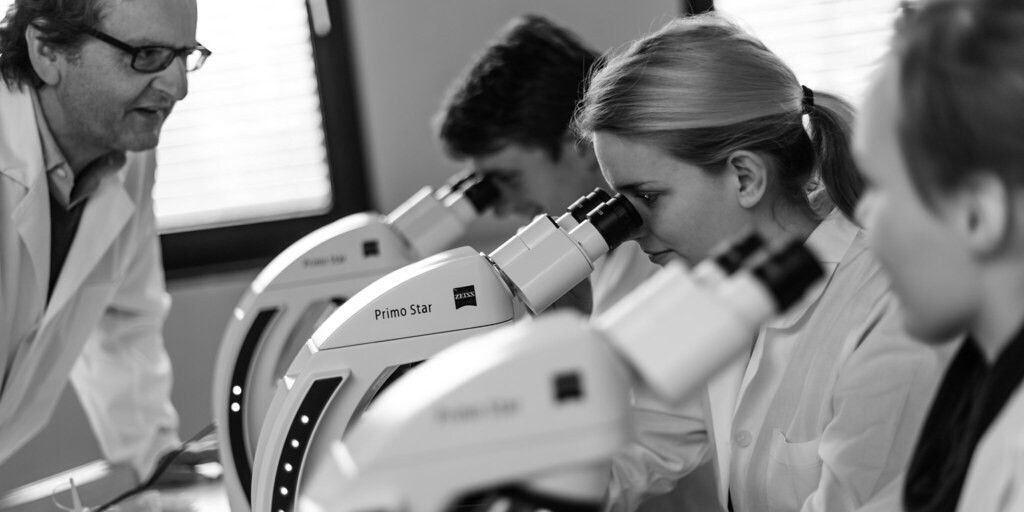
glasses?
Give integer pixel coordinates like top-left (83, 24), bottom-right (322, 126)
top-left (70, 21), bottom-right (212, 73)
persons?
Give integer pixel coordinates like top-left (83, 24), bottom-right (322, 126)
top-left (852, 0), bottom-right (1024, 512)
top-left (1, 1), bottom-right (227, 512)
top-left (438, 15), bottom-right (616, 314)
top-left (577, 13), bottom-right (946, 512)
